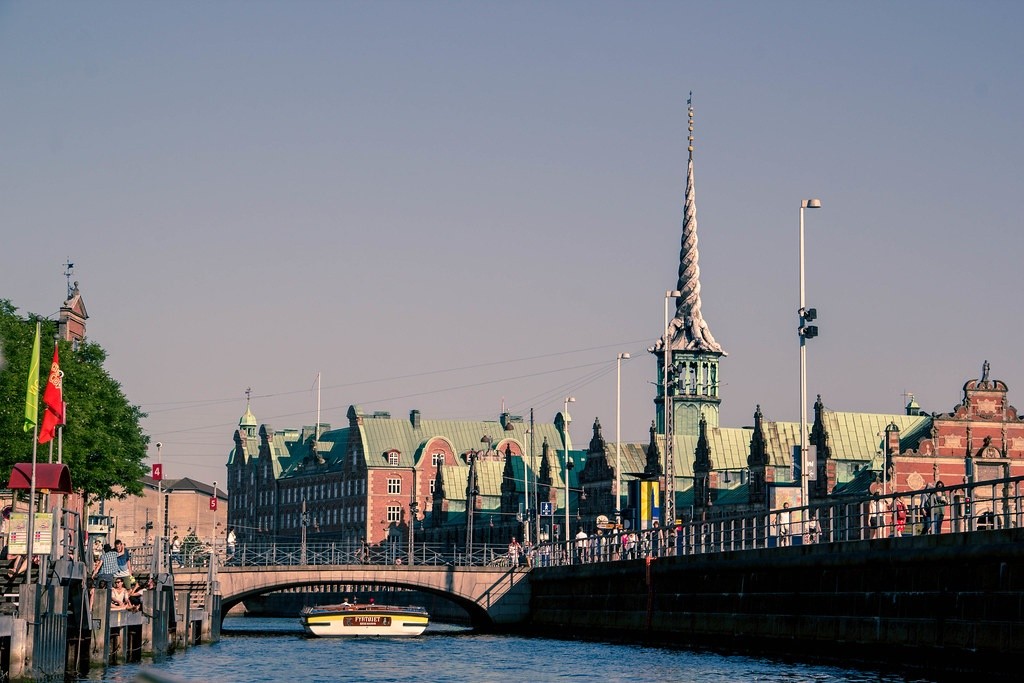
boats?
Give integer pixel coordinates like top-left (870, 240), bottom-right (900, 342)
top-left (301, 598), bottom-right (428, 636)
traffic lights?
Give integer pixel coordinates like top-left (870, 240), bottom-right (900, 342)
top-left (749, 470), bottom-right (755, 485)
top-left (965, 497), bottom-right (971, 516)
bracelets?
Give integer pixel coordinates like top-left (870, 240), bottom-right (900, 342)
top-left (367, 556), bottom-right (369, 557)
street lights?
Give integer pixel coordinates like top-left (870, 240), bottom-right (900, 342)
top-left (524, 428), bottom-right (531, 546)
top-left (212, 482), bottom-right (217, 569)
top-left (564, 396), bottom-right (575, 563)
top-left (156, 441), bottom-right (162, 572)
top-left (616, 351), bottom-right (630, 532)
top-left (877, 431), bottom-right (886, 495)
top-left (798, 195), bottom-right (824, 541)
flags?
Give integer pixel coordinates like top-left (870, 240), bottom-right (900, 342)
top-left (23, 321), bottom-right (39, 431)
top-left (39, 342), bottom-right (64, 445)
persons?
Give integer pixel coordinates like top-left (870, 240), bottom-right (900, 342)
top-left (808, 513), bottom-right (822, 543)
top-left (919, 480), bottom-right (948, 536)
top-left (92, 544), bottom-right (125, 604)
top-left (223, 526), bottom-right (237, 565)
top-left (576, 521), bottom-right (678, 564)
top-left (112, 539), bottom-right (131, 590)
top-left (537, 539), bottom-right (552, 566)
top-left (773, 502), bottom-right (793, 546)
top-left (0, 534), bottom-right (40, 569)
top-left (509, 537), bottom-right (534, 567)
top-left (200, 542), bottom-right (216, 567)
top-left (87, 577), bottom-right (156, 614)
top-left (354, 538), bottom-right (370, 564)
top-left (164, 536), bottom-right (185, 569)
top-left (93, 540), bottom-right (102, 560)
top-left (868, 488), bottom-right (909, 538)
